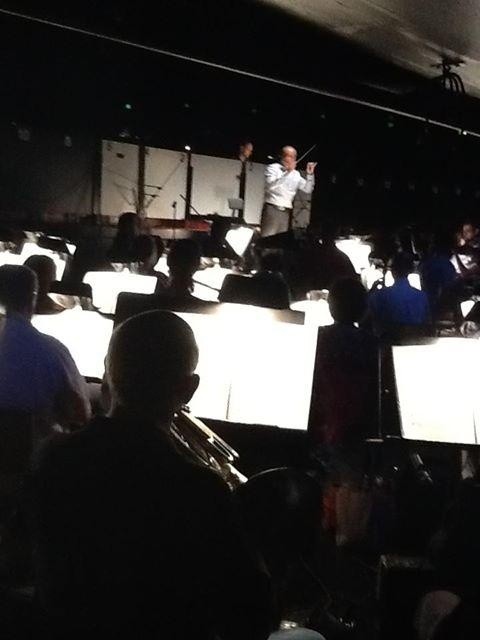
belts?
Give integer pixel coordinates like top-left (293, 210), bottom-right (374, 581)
top-left (264, 201), bottom-right (294, 214)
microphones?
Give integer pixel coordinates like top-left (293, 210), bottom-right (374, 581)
top-left (183, 144), bottom-right (193, 154)
top-left (172, 201), bottom-right (177, 207)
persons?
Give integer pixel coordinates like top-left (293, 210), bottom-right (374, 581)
top-left (231, 136), bottom-right (253, 162)
top-left (260, 144), bottom-right (318, 238)
